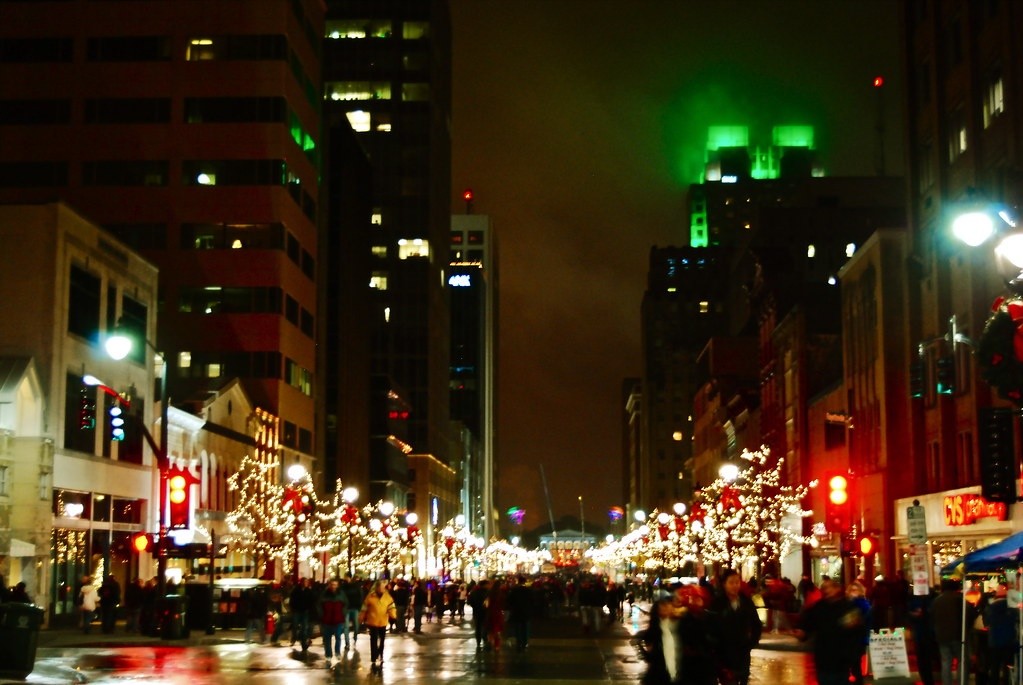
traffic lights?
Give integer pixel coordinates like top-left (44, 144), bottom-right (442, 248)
top-left (910, 363), bottom-right (924, 398)
top-left (826, 474), bottom-right (851, 534)
top-left (133, 534), bottom-right (154, 551)
top-left (108, 407), bottom-right (127, 442)
top-left (937, 359), bottom-right (955, 395)
top-left (81, 386), bottom-right (96, 430)
top-left (840, 537), bottom-right (878, 556)
top-left (169, 476), bottom-right (190, 530)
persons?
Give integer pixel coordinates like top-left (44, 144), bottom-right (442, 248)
top-left (78, 570), bottom-right (1023, 685)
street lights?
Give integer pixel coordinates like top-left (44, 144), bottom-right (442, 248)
top-left (286, 465), bottom-right (309, 585)
top-left (578, 495), bottom-right (585, 541)
top-left (405, 512), bottom-right (485, 581)
top-left (84, 328), bottom-right (170, 593)
top-left (380, 502), bottom-right (395, 579)
top-left (341, 487), bottom-right (359, 582)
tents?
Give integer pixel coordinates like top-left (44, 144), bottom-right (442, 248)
top-left (940, 530), bottom-right (1022, 684)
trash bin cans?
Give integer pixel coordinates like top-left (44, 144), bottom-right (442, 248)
top-left (0, 603), bottom-right (46, 681)
